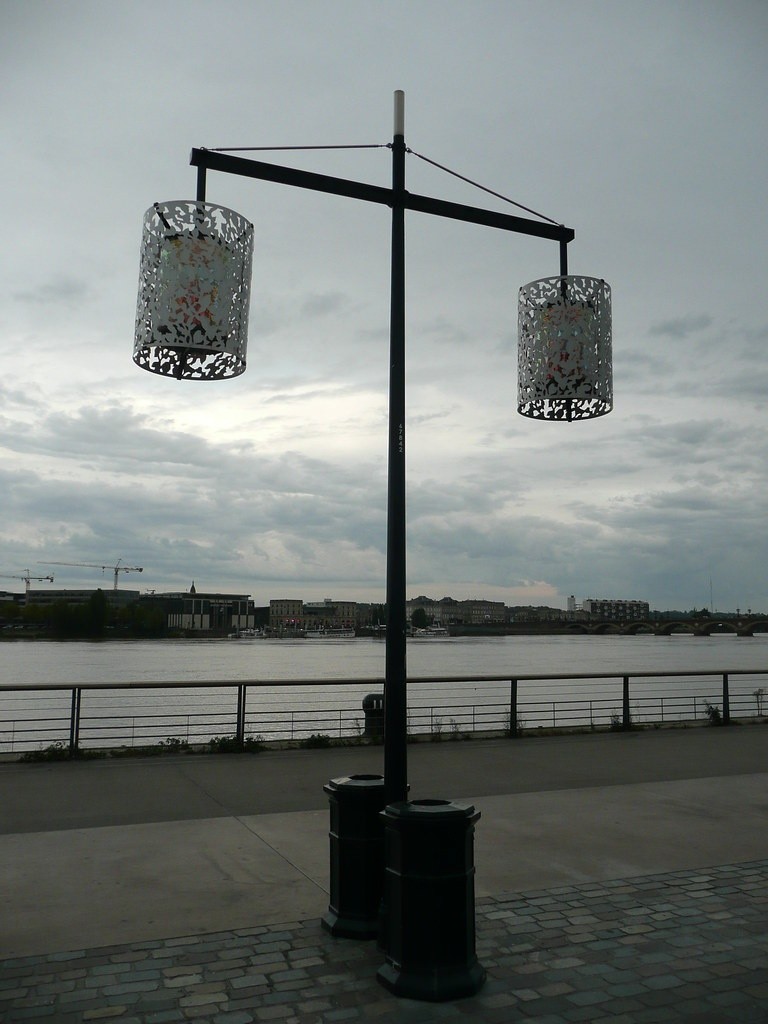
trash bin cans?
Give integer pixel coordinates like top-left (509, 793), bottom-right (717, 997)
top-left (322, 773), bottom-right (410, 941)
top-left (376, 797), bottom-right (486, 1004)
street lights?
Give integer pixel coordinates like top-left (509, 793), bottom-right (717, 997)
top-left (133, 150), bottom-right (613, 996)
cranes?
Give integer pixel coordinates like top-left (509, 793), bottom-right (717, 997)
top-left (36, 557), bottom-right (143, 596)
top-left (0, 568), bottom-right (54, 623)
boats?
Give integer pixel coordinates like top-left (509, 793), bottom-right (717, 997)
top-left (228, 628), bottom-right (269, 638)
top-left (299, 624), bottom-right (356, 637)
top-left (412, 623), bottom-right (450, 637)
